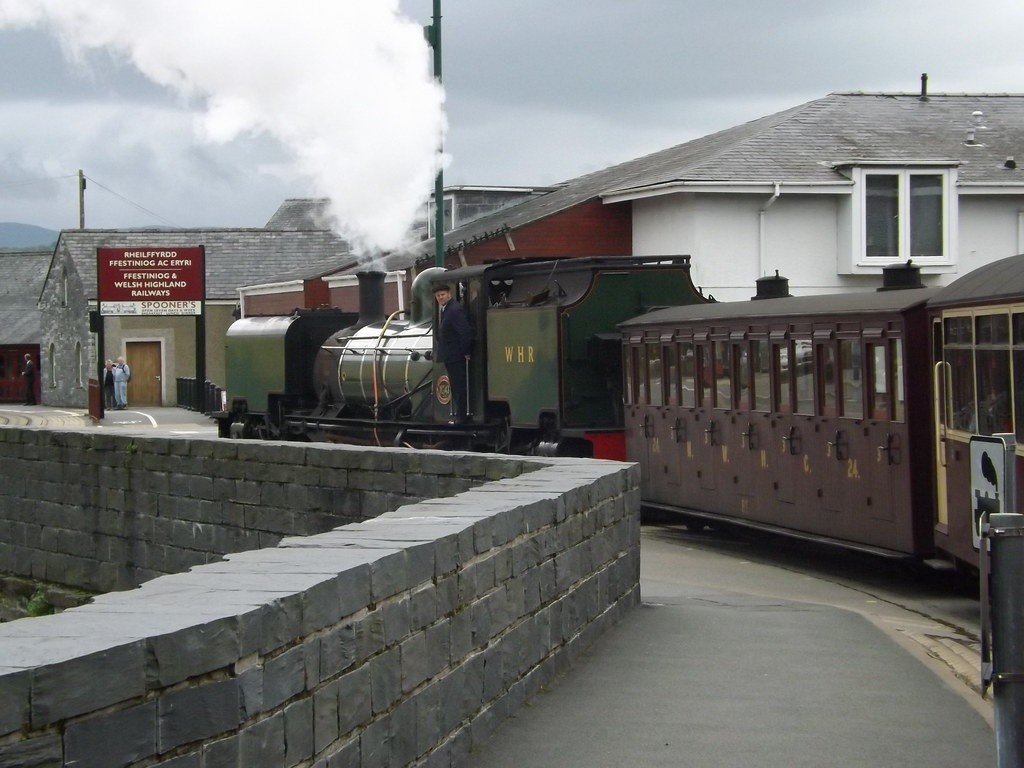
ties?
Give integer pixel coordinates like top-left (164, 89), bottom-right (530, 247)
top-left (440, 309), bottom-right (443, 324)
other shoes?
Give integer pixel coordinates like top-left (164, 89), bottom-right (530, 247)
top-left (448, 411), bottom-right (474, 426)
top-left (104, 404), bottom-right (129, 410)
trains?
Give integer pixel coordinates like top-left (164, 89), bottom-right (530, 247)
top-left (210, 252), bottom-right (1023, 768)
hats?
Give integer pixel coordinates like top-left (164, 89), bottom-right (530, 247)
top-left (432, 285), bottom-right (451, 293)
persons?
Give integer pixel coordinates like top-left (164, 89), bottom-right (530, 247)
top-left (104, 360), bottom-right (118, 410)
top-left (851, 341), bottom-right (861, 379)
top-left (113, 357), bottom-right (130, 409)
top-left (21, 354), bottom-right (37, 405)
top-left (433, 285), bottom-right (473, 425)
